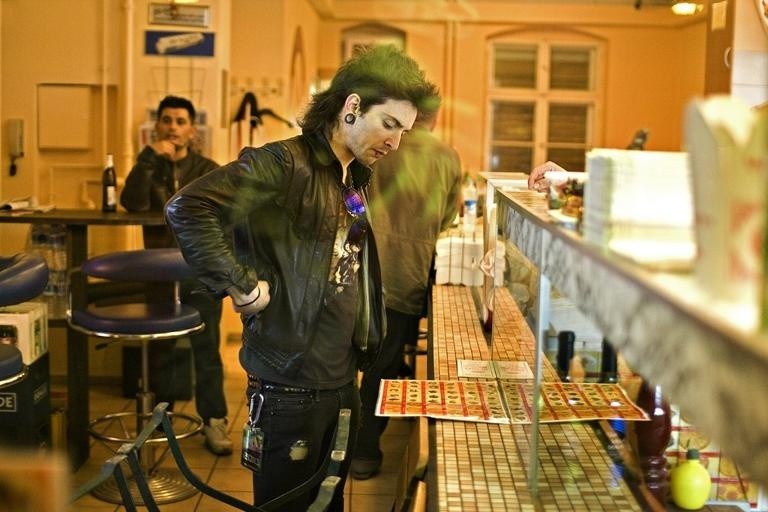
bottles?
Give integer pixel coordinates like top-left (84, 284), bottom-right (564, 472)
top-left (625, 127), bottom-right (649, 151)
top-left (463, 180), bottom-right (476, 230)
top-left (555, 331), bottom-right (575, 385)
top-left (27, 227), bottom-right (71, 320)
top-left (594, 338), bottom-right (626, 468)
top-left (100, 152), bottom-right (119, 214)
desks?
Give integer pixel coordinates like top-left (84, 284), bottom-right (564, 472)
top-left (4, 206), bottom-right (175, 474)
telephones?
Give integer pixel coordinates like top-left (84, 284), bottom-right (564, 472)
top-left (7, 118), bottom-right (24, 158)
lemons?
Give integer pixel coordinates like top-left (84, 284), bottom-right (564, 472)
top-left (669, 459), bottom-right (711, 510)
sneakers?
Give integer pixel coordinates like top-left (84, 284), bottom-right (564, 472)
top-left (204, 416), bottom-right (232, 452)
top-left (352, 454), bottom-right (383, 480)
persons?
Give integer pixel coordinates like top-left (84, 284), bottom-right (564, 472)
top-left (161, 40), bottom-right (440, 512)
top-left (529, 159), bottom-right (584, 197)
top-left (348, 82), bottom-right (463, 482)
top-left (118, 95), bottom-right (235, 456)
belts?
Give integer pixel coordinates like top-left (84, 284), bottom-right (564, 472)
top-left (247, 382), bottom-right (352, 402)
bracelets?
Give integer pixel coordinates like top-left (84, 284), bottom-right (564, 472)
top-left (233, 285), bottom-right (262, 310)
